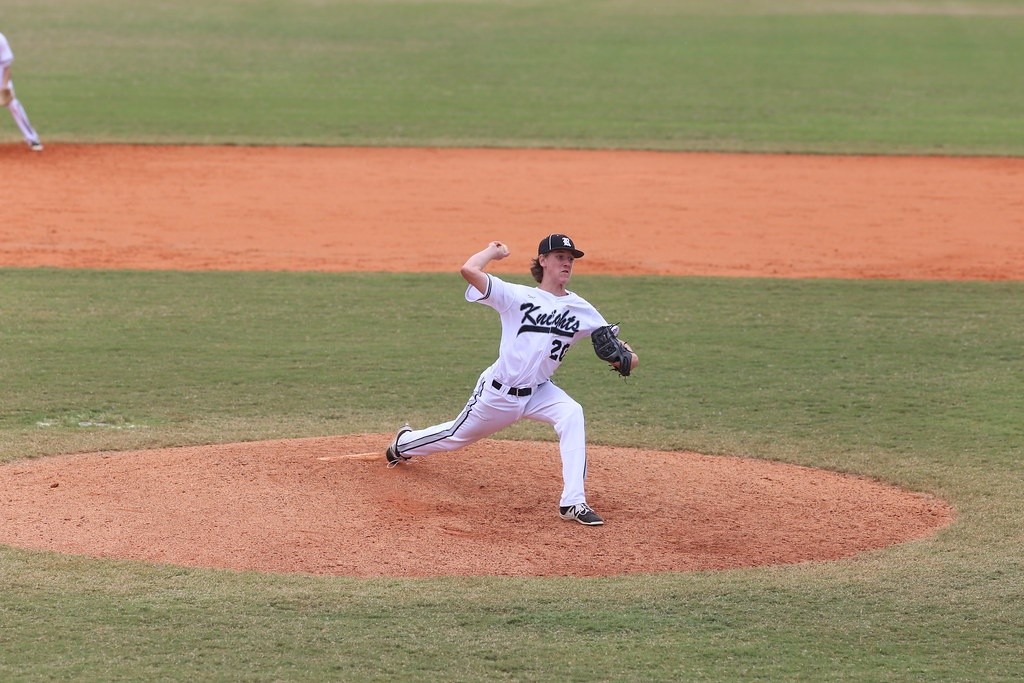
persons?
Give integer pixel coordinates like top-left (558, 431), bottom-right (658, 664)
top-left (386, 232), bottom-right (638, 525)
top-left (1, 32), bottom-right (43, 151)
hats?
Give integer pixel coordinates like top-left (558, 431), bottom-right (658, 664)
top-left (538, 234), bottom-right (584, 259)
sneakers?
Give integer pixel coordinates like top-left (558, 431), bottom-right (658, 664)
top-left (385, 427), bottom-right (413, 464)
top-left (560, 502), bottom-right (604, 526)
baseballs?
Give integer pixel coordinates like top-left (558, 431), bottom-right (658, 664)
top-left (499, 243), bottom-right (507, 254)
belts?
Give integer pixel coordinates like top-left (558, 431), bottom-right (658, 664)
top-left (492, 380), bottom-right (531, 397)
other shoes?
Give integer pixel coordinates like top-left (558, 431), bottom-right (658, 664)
top-left (29, 141), bottom-right (44, 152)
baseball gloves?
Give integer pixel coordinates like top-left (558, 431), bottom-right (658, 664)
top-left (0, 88), bottom-right (13, 107)
top-left (591, 323), bottom-right (631, 377)
